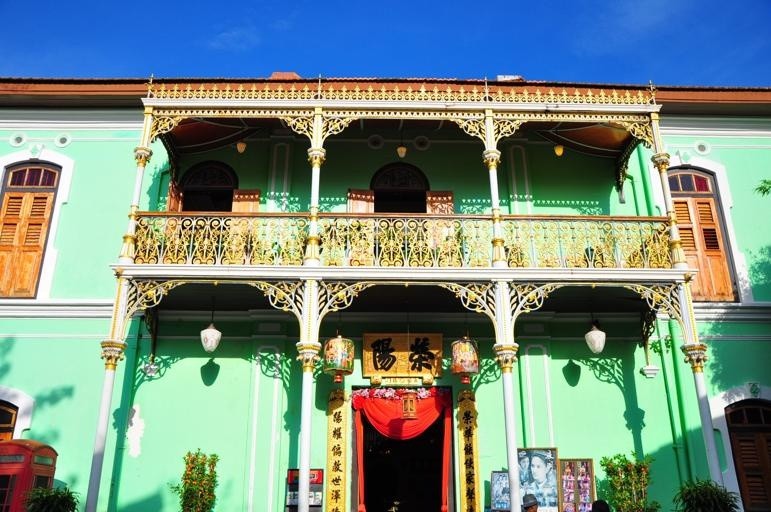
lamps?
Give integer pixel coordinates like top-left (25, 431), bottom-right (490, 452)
top-left (396, 127), bottom-right (407, 158)
top-left (322, 306), bottom-right (354, 385)
top-left (199, 286), bottom-right (221, 352)
top-left (450, 301), bottom-right (481, 384)
top-left (236, 129), bottom-right (246, 154)
top-left (552, 130), bottom-right (564, 156)
top-left (401, 288), bottom-right (416, 421)
top-left (584, 298), bottom-right (607, 356)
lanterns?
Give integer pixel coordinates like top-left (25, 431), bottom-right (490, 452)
top-left (323, 330), bottom-right (355, 383)
top-left (451, 336), bottom-right (480, 384)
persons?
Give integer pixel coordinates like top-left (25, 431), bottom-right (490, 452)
top-left (519, 450), bottom-right (531, 488)
top-left (530, 449), bottom-right (556, 492)
top-left (593, 500), bottom-right (611, 512)
top-left (521, 492), bottom-right (540, 512)
